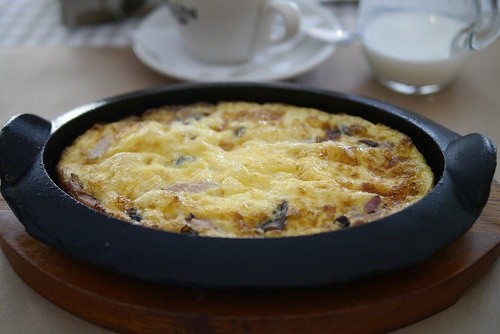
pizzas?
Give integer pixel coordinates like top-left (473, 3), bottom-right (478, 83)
top-left (55, 100), bottom-right (434, 238)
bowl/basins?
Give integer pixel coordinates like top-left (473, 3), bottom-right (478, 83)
top-left (0, 80), bottom-right (498, 302)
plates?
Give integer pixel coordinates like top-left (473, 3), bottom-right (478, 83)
top-left (131, 1), bottom-right (344, 82)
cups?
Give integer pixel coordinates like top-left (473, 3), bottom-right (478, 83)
top-left (358, 1), bottom-right (500, 96)
top-left (168, 1), bottom-right (302, 66)
